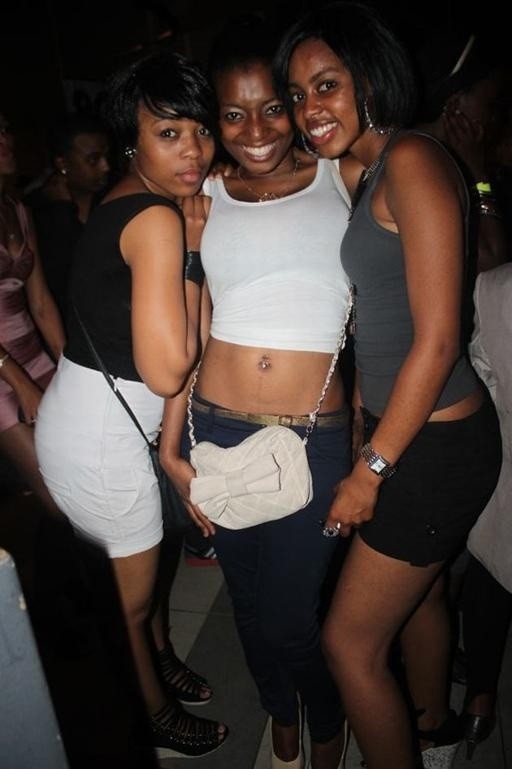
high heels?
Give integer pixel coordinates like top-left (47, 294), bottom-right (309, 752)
top-left (153, 648), bottom-right (215, 706)
top-left (459, 708), bottom-right (498, 761)
top-left (137, 708), bottom-right (229, 761)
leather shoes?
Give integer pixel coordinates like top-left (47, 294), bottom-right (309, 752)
top-left (268, 707), bottom-right (305, 769)
top-left (308, 718), bottom-right (346, 769)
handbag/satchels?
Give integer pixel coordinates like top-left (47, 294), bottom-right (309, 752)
top-left (148, 443), bottom-right (193, 539)
top-left (188, 423), bottom-right (313, 532)
